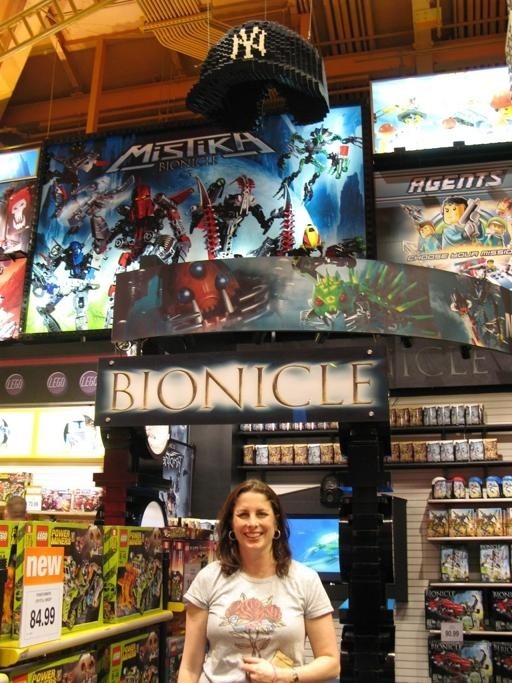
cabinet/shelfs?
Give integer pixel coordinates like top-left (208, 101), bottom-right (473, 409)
top-left (424, 495), bottom-right (512, 681)
top-left (228, 423), bottom-right (512, 479)
top-left (0, 424), bottom-right (186, 683)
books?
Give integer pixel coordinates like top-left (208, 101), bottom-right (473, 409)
top-left (25, 487), bottom-right (42, 512)
top-left (19, 546), bottom-right (65, 648)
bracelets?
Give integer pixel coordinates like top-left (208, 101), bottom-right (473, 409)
top-left (272, 665), bottom-right (277, 683)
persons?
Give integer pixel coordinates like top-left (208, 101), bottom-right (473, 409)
top-left (3, 496), bottom-right (27, 521)
top-left (177, 479), bottom-right (341, 683)
top-left (94, 496), bottom-right (104, 525)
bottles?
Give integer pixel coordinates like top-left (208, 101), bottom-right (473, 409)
top-left (239, 405), bottom-right (500, 463)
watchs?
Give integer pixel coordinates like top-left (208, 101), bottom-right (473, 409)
top-left (289, 668), bottom-right (299, 683)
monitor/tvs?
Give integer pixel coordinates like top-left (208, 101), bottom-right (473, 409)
top-left (284, 513), bottom-right (342, 583)
top-left (370, 66), bottom-right (512, 155)
